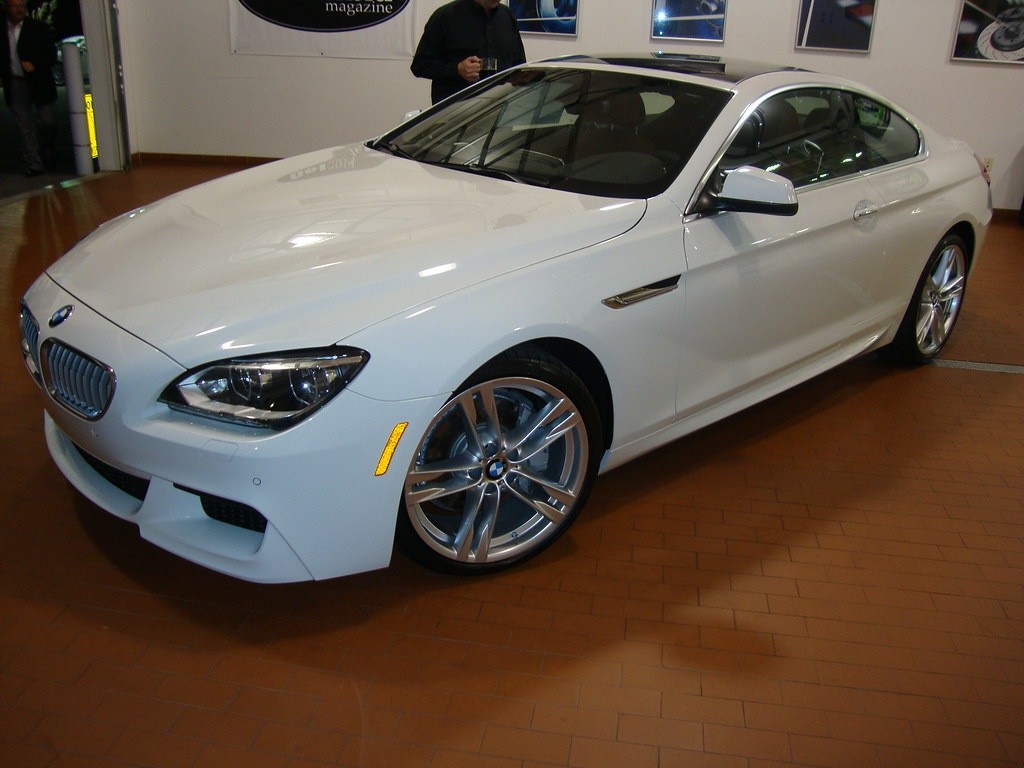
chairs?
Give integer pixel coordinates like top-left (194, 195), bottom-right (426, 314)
top-left (563, 85), bottom-right (652, 161)
top-left (733, 97), bottom-right (800, 150)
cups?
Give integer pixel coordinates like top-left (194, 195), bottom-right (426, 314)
top-left (475, 58), bottom-right (497, 80)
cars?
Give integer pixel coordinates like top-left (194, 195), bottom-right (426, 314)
top-left (17, 54), bottom-right (995, 583)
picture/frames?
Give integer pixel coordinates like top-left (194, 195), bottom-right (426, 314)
top-left (650, 0), bottom-right (727, 44)
top-left (793, 0), bottom-right (879, 55)
top-left (948, 0), bottom-right (1024, 65)
top-left (508, 0), bottom-right (580, 37)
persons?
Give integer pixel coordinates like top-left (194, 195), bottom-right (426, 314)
top-left (0, 0), bottom-right (60, 178)
top-left (410, 0), bottom-right (528, 107)
